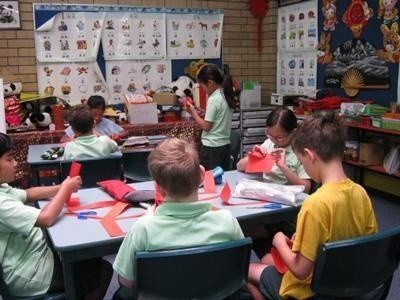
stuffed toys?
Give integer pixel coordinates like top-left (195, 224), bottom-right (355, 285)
top-left (3, 82), bottom-right (23, 98)
top-left (170, 74), bottom-right (196, 100)
top-left (21, 102), bottom-right (52, 127)
top-left (108, 110), bottom-right (127, 124)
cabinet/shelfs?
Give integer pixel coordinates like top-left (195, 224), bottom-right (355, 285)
top-left (340, 120), bottom-right (400, 186)
top-left (242, 103), bottom-right (281, 159)
top-left (227, 105), bottom-right (241, 160)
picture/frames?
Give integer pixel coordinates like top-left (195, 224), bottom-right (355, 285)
top-left (0, 0), bottom-right (21, 30)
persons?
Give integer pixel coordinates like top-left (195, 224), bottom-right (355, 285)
top-left (246, 115), bottom-right (378, 300)
top-left (112, 137), bottom-right (246, 300)
top-left (183, 65), bottom-right (235, 171)
top-left (0, 133), bottom-right (100, 300)
top-left (60, 96), bottom-right (129, 143)
top-left (61, 107), bottom-right (119, 160)
top-left (236, 107), bottom-right (312, 194)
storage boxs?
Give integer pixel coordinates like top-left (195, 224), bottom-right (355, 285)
top-left (124, 94), bottom-right (160, 125)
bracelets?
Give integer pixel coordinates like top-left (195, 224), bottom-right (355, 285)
top-left (189, 105), bottom-right (194, 113)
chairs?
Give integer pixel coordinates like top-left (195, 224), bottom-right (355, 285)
top-left (5, 129), bottom-right (400, 300)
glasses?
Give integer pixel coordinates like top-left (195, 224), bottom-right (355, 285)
top-left (264, 127), bottom-right (292, 142)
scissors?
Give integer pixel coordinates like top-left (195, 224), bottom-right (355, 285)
top-left (64, 211), bottom-right (97, 220)
top-left (247, 203), bottom-right (281, 209)
